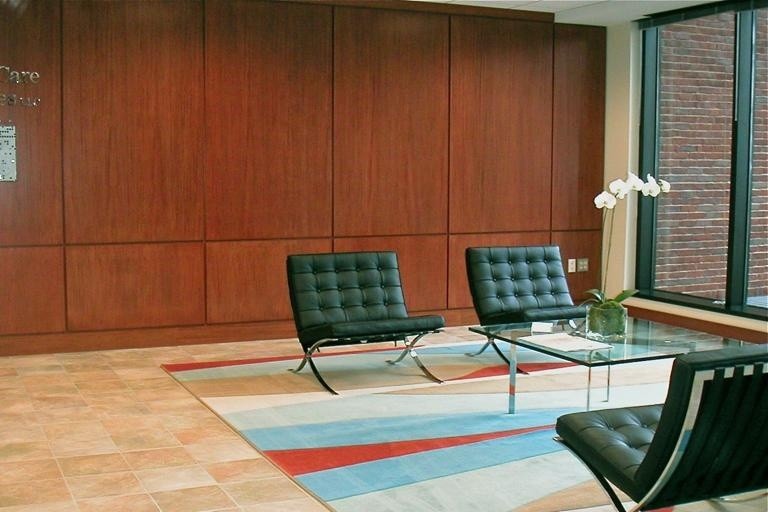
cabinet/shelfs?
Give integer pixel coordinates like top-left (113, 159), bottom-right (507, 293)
top-left (1, 0), bottom-right (66, 338)
top-left (447, 14), bottom-right (554, 310)
top-left (63, 0), bottom-right (207, 334)
top-left (550, 23), bottom-right (607, 304)
top-left (204, 0), bottom-right (333, 326)
top-left (333, 13), bottom-right (450, 313)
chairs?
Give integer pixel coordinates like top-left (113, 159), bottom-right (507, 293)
top-left (552, 343), bottom-right (767, 512)
top-left (287, 250), bottom-right (445, 395)
top-left (464, 245), bottom-right (616, 376)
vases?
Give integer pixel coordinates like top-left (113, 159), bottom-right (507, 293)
top-left (585, 305), bottom-right (628, 340)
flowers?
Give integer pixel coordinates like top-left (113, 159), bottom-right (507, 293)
top-left (575, 172), bottom-right (671, 335)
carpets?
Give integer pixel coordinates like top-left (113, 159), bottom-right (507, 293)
top-left (159, 338), bottom-right (675, 512)
